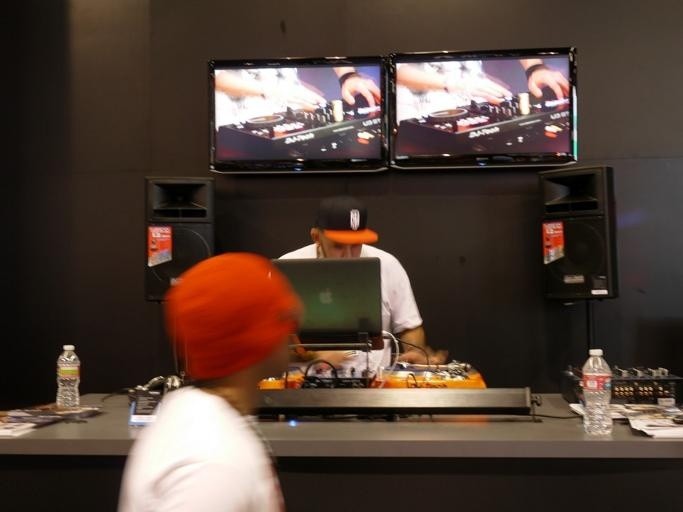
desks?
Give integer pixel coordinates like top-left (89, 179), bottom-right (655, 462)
top-left (1, 391), bottom-right (682, 512)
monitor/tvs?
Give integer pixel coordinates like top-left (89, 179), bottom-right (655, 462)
top-left (390, 48), bottom-right (578, 170)
top-left (208, 55), bottom-right (389, 174)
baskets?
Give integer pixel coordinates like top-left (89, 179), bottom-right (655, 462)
top-left (561, 362), bottom-right (683, 404)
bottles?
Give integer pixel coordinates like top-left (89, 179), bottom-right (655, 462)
top-left (582, 349), bottom-right (617, 436)
top-left (53, 345), bottom-right (82, 408)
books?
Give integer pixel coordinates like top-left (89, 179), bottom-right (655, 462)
top-left (0, 403), bottom-right (104, 440)
top-left (570, 399), bottom-right (683, 440)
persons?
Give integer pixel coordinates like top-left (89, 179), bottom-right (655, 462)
top-left (266, 190), bottom-right (426, 375)
top-left (114, 247), bottom-right (308, 511)
top-left (391, 57), bottom-right (570, 130)
top-left (215, 61), bottom-right (379, 124)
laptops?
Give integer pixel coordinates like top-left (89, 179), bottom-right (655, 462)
top-left (270, 258), bottom-right (384, 351)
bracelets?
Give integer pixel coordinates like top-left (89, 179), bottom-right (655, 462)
top-left (520, 61), bottom-right (551, 77)
top-left (333, 69), bottom-right (362, 87)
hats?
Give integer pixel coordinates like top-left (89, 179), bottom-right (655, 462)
top-left (315, 196), bottom-right (378, 245)
top-left (167, 253), bottom-right (301, 381)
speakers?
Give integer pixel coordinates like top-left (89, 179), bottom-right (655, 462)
top-left (539, 167), bottom-right (619, 303)
top-left (144, 175), bottom-right (216, 304)
